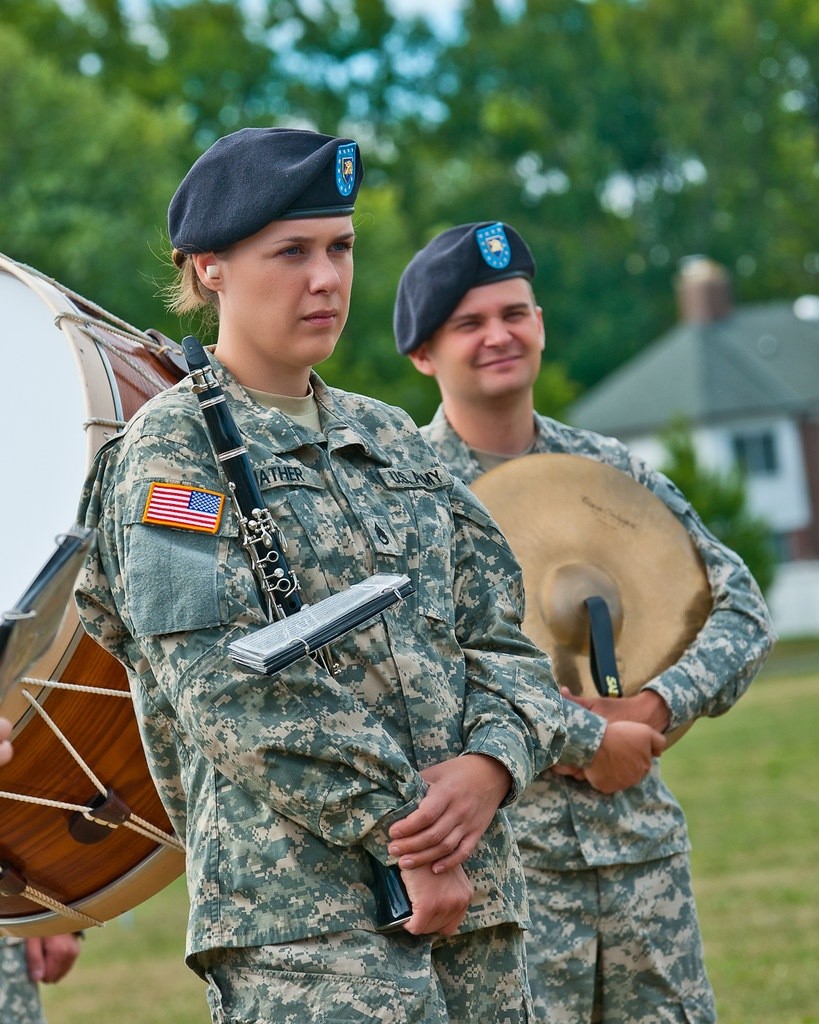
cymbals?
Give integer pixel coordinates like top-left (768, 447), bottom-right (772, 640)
top-left (464, 451), bottom-right (711, 754)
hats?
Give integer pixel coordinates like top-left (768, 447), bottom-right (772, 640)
top-left (167, 128), bottom-right (363, 253)
top-left (395, 221), bottom-right (537, 356)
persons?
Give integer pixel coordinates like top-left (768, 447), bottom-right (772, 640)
top-left (393, 221), bottom-right (776, 1024)
top-left (71, 125), bottom-right (568, 1024)
top-left (0, 714), bottom-right (85, 1024)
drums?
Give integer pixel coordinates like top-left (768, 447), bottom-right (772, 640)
top-left (0, 253), bottom-right (190, 938)
